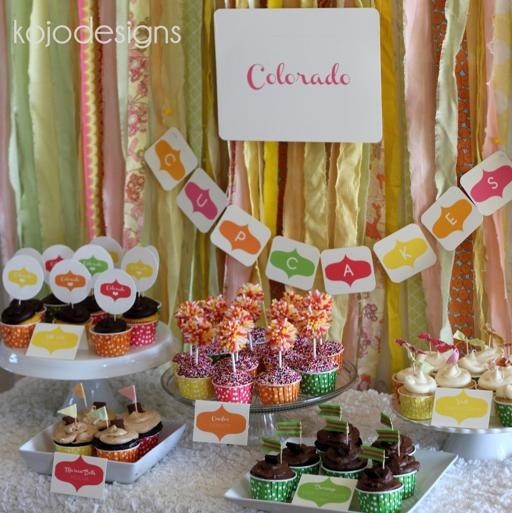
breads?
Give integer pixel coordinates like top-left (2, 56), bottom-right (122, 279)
top-left (392, 346), bottom-right (512, 427)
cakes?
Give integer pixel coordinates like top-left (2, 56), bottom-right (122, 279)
top-left (172, 324), bottom-right (345, 405)
top-left (250, 423), bottom-right (420, 513)
top-left (53, 401), bottom-right (163, 463)
top-left (1, 292), bottom-right (161, 357)
